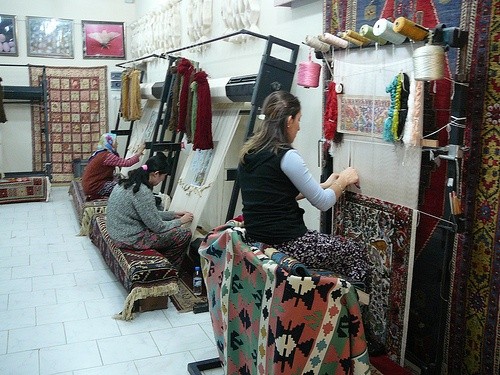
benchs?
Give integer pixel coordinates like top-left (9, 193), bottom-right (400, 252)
top-left (187, 216), bottom-right (368, 375)
top-left (88, 213), bottom-right (182, 321)
top-left (68, 177), bottom-right (109, 236)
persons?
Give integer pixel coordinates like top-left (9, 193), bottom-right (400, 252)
top-left (105, 157), bottom-right (194, 270)
top-left (82, 134), bottom-right (145, 195)
top-left (238, 91), bottom-right (386, 356)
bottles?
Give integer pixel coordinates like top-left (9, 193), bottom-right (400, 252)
top-left (193, 267), bottom-right (202, 296)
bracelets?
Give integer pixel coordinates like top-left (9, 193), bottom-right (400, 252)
top-left (334, 182), bottom-right (344, 193)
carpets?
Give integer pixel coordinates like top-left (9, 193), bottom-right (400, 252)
top-left (169, 257), bottom-right (209, 313)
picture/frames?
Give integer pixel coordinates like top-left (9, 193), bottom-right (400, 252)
top-left (0, 13), bottom-right (20, 57)
top-left (81, 20), bottom-right (127, 60)
top-left (25, 16), bottom-right (74, 59)
